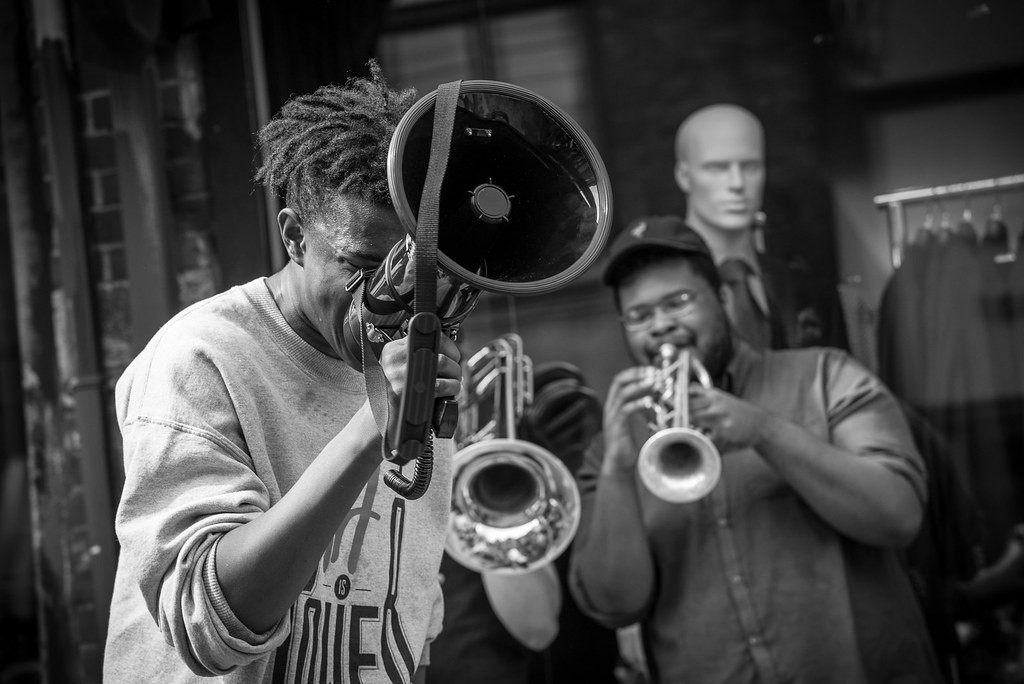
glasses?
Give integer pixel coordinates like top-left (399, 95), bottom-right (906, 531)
top-left (618, 286), bottom-right (709, 332)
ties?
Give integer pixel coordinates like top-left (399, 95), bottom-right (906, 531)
top-left (722, 258), bottom-right (771, 349)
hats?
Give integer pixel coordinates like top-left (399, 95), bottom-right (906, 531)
top-left (603, 216), bottom-right (716, 287)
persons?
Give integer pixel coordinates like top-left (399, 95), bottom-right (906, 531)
top-left (670, 100), bottom-right (811, 350)
top-left (564, 209), bottom-right (941, 684)
top-left (426, 541), bottom-right (622, 684)
top-left (101, 55), bottom-right (466, 684)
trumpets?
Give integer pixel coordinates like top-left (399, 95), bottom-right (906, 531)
top-left (638, 337), bottom-right (725, 505)
top-left (439, 331), bottom-right (581, 578)
